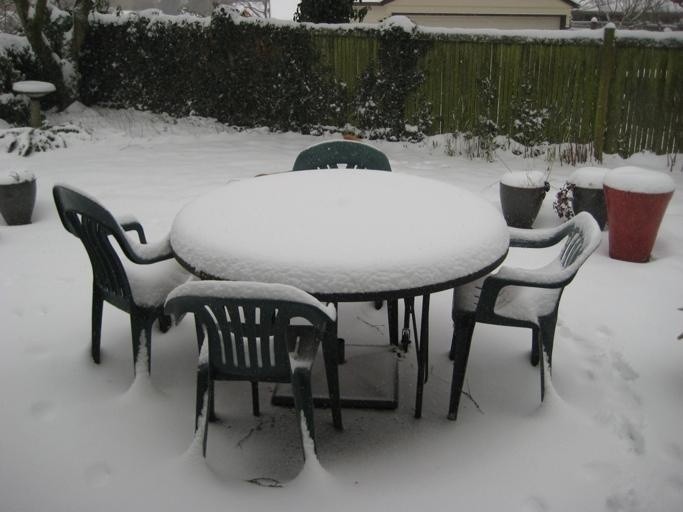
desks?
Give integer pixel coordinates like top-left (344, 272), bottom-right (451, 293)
top-left (165, 164), bottom-right (512, 422)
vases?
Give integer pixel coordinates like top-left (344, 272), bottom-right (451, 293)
top-left (568, 184), bottom-right (676, 263)
top-left (499, 181), bottom-right (552, 229)
top-left (0, 180), bottom-right (37, 226)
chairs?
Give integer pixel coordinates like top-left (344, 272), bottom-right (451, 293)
top-left (292, 138), bottom-right (393, 314)
top-left (149, 273), bottom-right (346, 458)
top-left (51, 181), bottom-right (202, 380)
top-left (444, 205), bottom-right (602, 424)
